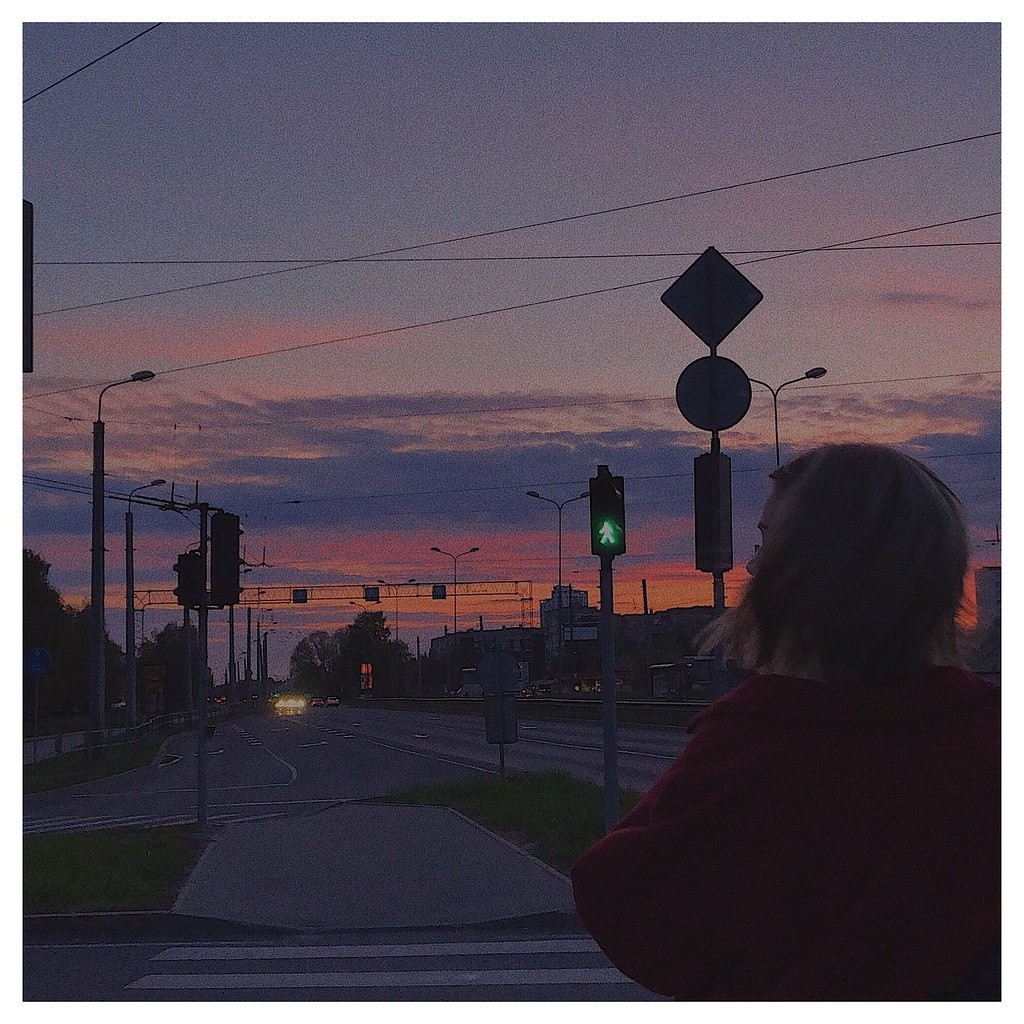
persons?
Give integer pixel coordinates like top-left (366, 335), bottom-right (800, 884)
top-left (575, 443), bottom-right (1001, 1002)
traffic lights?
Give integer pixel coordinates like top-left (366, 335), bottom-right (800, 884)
top-left (209, 510), bottom-right (243, 606)
top-left (590, 477), bottom-right (627, 557)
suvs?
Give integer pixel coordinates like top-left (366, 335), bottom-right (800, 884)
top-left (272, 695), bottom-right (340, 708)
top-left (678, 656), bottom-right (714, 701)
top-left (523, 676), bottom-right (604, 702)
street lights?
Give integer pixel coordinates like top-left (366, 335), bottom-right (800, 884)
top-left (349, 600), bottom-right (381, 611)
top-left (524, 490), bottom-right (591, 675)
top-left (748, 366), bottom-right (827, 467)
top-left (227, 568), bottom-right (277, 701)
top-left (125, 481), bottom-right (167, 726)
top-left (376, 579), bottom-right (416, 641)
top-left (89, 370), bottom-right (158, 726)
top-left (430, 546), bottom-right (481, 696)
